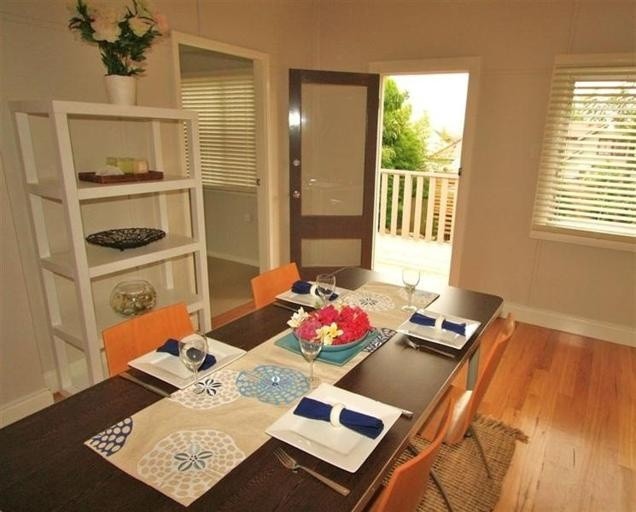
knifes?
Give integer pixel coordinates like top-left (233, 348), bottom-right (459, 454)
top-left (117, 371), bottom-right (171, 399)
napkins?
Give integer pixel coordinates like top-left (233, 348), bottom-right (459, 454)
top-left (293, 396), bottom-right (384, 440)
top-left (409, 311), bottom-right (466, 337)
top-left (156, 338), bottom-right (217, 372)
top-left (291, 279), bottom-right (338, 301)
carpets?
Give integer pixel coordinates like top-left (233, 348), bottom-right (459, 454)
top-left (381, 411), bottom-right (529, 511)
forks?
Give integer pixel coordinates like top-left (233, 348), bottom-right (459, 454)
top-left (400, 335), bottom-right (455, 360)
top-left (272, 446), bottom-right (352, 499)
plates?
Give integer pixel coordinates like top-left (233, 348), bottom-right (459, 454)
top-left (273, 281), bottom-right (356, 310)
top-left (126, 333), bottom-right (247, 391)
top-left (394, 308), bottom-right (482, 351)
top-left (263, 382), bottom-right (393, 474)
top-left (83, 226), bottom-right (167, 253)
top-left (291, 325), bottom-right (369, 353)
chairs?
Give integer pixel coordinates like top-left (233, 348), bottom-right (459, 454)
top-left (250, 262), bottom-right (301, 310)
top-left (102, 301), bottom-right (195, 378)
top-left (365, 397), bottom-right (456, 512)
top-left (406, 312), bottom-right (517, 512)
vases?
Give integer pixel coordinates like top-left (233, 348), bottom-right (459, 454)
top-left (103, 76), bottom-right (137, 106)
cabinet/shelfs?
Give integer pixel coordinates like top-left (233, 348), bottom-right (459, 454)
top-left (6, 98), bottom-right (213, 397)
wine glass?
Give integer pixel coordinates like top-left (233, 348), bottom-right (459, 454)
top-left (176, 331), bottom-right (210, 393)
top-left (315, 273), bottom-right (336, 308)
top-left (297, 327), bottom-right (324, 387)
top-left (401, 267), bottom-right (420, 313)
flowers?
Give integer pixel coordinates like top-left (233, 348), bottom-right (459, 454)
top-left (287, 302), bottom-right (373, 346)
top-left (67, 1), bottom-right (168, 74)
